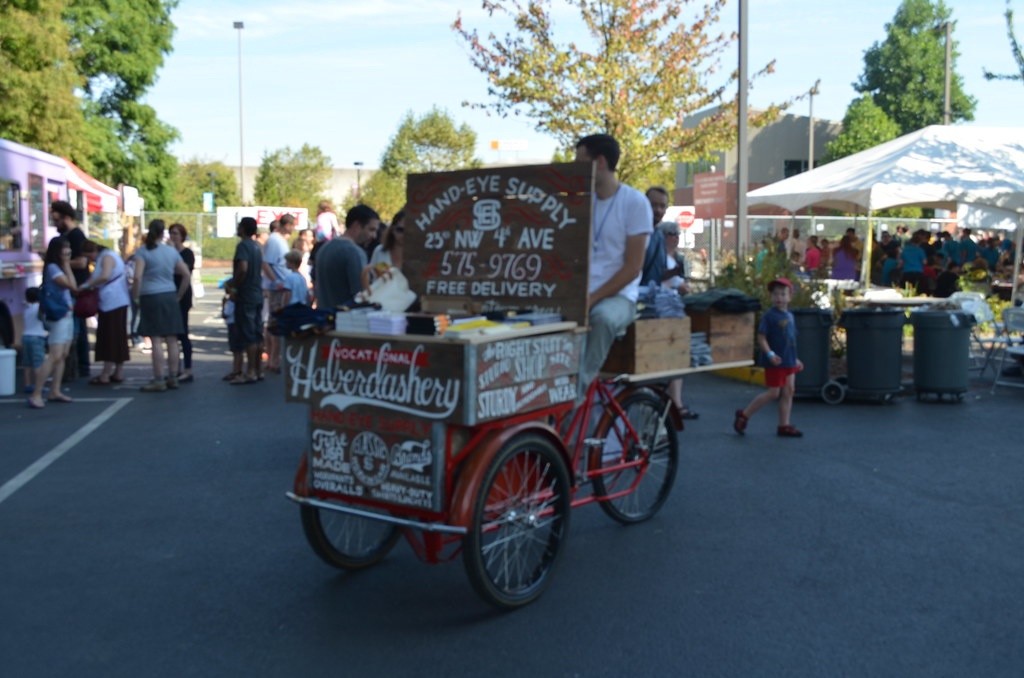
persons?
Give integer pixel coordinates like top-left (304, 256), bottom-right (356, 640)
top-left (766, 351), bottom-right (775, 359)
top-left (552, 134), bottom-right (700, 438)
top-left (754, 224), bottom-right (1015, 298)
top-left (734, 279), bottom-right (804, 437)
top-left (20, 201), bottom-right (195, 409)
top-left (223, 201), bottom-right (407, 384)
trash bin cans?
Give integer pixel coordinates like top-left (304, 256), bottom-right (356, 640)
top-left (910, 312), bottom-right (977, 391)
top-left (790, 309), bottom-right (831, 391)
top-left (840, 310), bottom-right (906, 390)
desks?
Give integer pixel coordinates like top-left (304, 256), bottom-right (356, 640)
top-left (844, 296), bottom-right (959, 310)
top-left (803, 278), bottom-right (859, 354)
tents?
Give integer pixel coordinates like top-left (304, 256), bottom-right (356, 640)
top-left (745, 124), bottom-right (1024, 283)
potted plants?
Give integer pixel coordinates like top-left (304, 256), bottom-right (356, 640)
top-left (710, 233), bottom-right (821, 388)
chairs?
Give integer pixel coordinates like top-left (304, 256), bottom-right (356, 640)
top-left (804, 278), bottom-right (1024, 395)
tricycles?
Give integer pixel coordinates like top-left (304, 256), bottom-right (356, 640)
top-left (285, 161), bottom-right (754, 609)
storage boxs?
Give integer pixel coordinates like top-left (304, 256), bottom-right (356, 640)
top-left (685, 307), bottom-right (755, 364)
top-left (600, 317), bottom-right (691, 374)
top-left (285, 318), bottom-right (580, 425)
